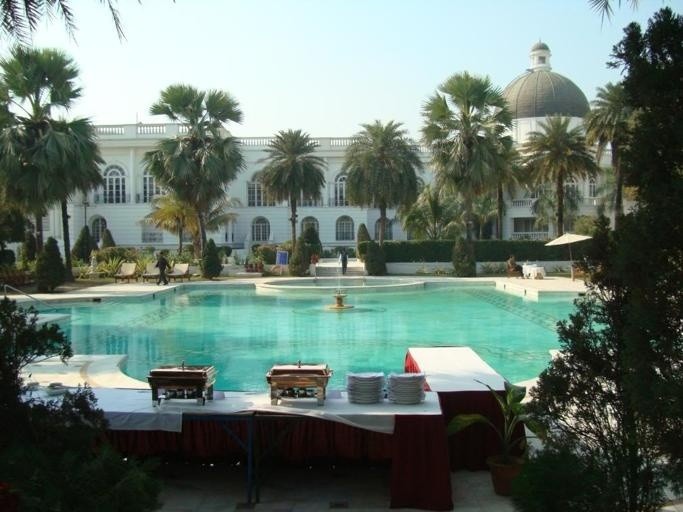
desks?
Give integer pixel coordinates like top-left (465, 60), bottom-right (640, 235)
top-left (407, 345), bottom-right (522, 463)
top-left (521, 263), bottom-right (545, 280)
top-left (18, 390), bottom-right (444, 505)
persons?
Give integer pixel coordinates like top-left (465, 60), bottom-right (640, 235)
top-left (154, 252), bottom-right (171, 285)
top-left (338, 248), bottom-right (348, 274)
top-left (507, 254), bottom-right (523, 279)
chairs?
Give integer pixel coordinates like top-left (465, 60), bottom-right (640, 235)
top-left (113, 261), bottom-right (191, 285)
top-left (505, 262), bottom-right (520, 279)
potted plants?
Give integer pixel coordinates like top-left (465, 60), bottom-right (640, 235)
top-left (445, 377), bottom-right (551, 498)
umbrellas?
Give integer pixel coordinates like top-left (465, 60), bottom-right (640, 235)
top-left (544, 232), bottom-right (593, 280)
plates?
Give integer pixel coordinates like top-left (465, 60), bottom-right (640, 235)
top-left (345, 372), bottom-right (427, 406)
